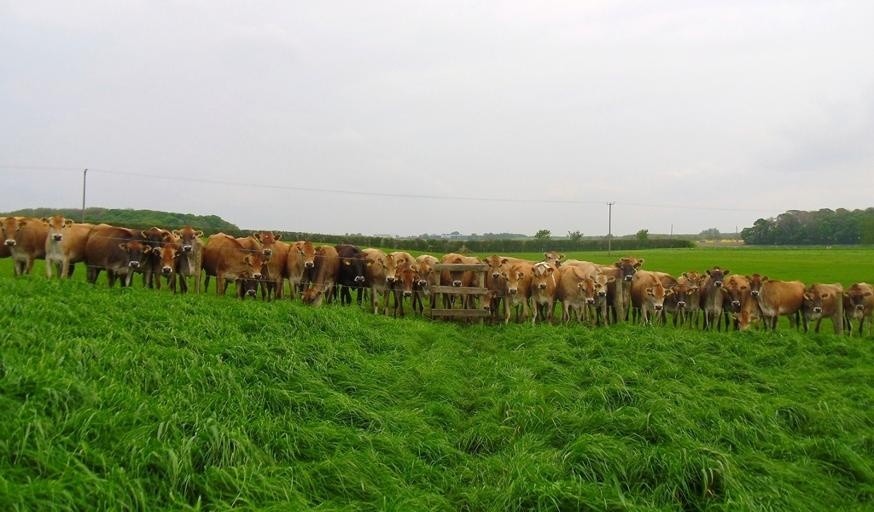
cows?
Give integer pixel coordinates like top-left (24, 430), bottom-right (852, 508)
top-left (0, 213), bottom-right (874, 336)
